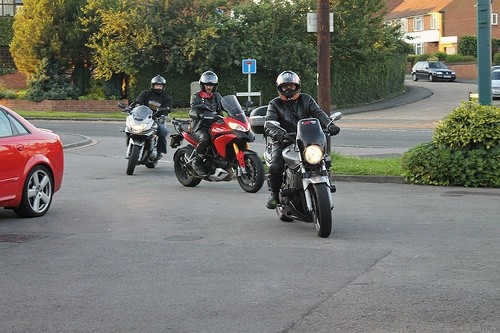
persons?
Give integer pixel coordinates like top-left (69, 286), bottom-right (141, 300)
top-left (121, 75), bottom-right (172, 160)
top-left (264, 70), bottom-right (340, 209)
top-left (189, 70), bottom-right (235, 177)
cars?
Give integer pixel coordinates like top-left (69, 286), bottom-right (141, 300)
top-left (491, 66), bottom-right (500, 100)
top-left (0, 104), bottom-right (64, 219)
top-left (411, 61), bottom-right (456, 82)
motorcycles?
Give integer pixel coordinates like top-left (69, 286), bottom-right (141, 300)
top-left (249, 105), bottom-right (343, 238)
top-left (169, 95), bottom-right (266, 193)
top-left (117, 102), bottom-right (170, 176)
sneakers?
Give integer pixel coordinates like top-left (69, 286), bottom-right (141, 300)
top-left (266, 192), bottom-right (280, 209)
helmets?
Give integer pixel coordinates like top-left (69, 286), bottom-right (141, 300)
top-left (151, 75), bottom-right (166, 93)
top-left (198, 71), bottom-right (218, 93)
top-left (275, 70), bottom-right (301, 101)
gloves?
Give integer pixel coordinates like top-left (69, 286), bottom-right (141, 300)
top-left (272, 130), bottom-right (285, 144)
top-left (202, 111), bottom-right (212, 117)
top-left (329, 124), bottom-right (340, 136)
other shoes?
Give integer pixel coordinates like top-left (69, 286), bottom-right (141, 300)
top-left (155, 154), bottom-right (164, 160)
top-left (192, 157), bottom-right (208, 177)
